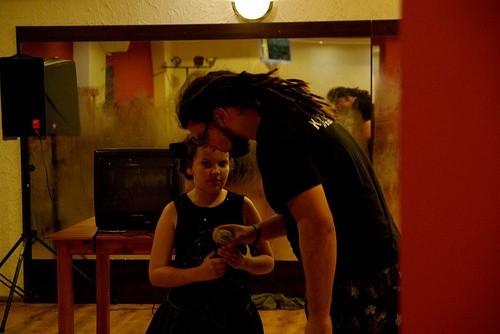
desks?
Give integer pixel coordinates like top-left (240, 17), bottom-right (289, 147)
top-left (45, 216), bottom-right (176, 334)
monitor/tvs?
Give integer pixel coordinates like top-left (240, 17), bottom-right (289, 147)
top-left (94, 146), bottom-right (186, 229)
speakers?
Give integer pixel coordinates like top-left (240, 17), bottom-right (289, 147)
top-left (0, 54), bottom-right (47, 140)
top-left (43, 58), bottom-right (81, 136)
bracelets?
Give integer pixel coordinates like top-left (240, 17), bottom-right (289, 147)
top-left (253, 225), bottom-right (260, 245)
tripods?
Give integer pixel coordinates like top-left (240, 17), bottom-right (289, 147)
top-left (0, 137), bottom-right (118, 333)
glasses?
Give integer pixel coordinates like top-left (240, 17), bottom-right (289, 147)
top-left (196, 119), bottom-right (212, 148)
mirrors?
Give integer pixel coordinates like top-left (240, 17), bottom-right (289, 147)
top-left (14, 21), bottom-right (374, 306)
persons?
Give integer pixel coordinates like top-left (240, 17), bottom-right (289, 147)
top-left (176, 70), bottom-right (401, 334)
top-left (144, 135), bottom-right (274, 334)
top-left (324, 87), bottom-right (373, 169)
